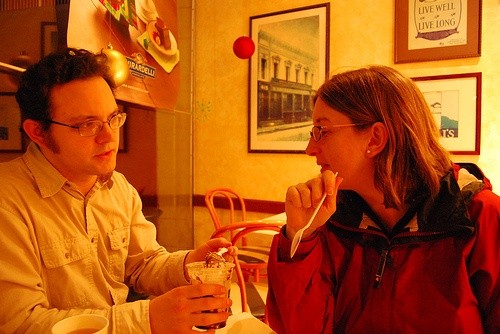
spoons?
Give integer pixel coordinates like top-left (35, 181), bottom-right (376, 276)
top-left (210, 247), bottom-right (228, 261)
top-left (290, 172), bottom-right (339, 259)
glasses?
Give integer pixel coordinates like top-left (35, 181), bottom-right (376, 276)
top-left (33, 111), bottom-right (126, 136)
top-left (309, 122), bottom-right (374, 141)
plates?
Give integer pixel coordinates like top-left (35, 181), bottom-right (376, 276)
top-left (135, 0), bottom-right (158, 24)
top-left (147, 21), bottom-right (178, 56)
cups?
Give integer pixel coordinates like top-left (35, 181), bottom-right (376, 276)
top-left (185, 261), bottom-right (235, 329)
top-left (51, 314), bottom-right (109, 334)
top-left (139, 0), bottom-right (155, 20)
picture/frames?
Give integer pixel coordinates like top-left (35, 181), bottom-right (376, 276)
top-left (394, 0), bottom-right (482, 63)
top-left (40, 21), bottom-right (58, 58)
top-left (0, 91), bottom-right (26, 154)
top-left (247, 2), bottom-right (330, 153)
top-left (410, 72), bottom-right (482, 155)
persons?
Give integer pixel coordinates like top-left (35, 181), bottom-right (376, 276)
top-left (264, 67), bottom-right (500, 334)
top-left (0, 47), bottom-right (237, 334)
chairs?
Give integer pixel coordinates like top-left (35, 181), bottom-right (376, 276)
top-left (204, 187), bottom-right (285, 323)
